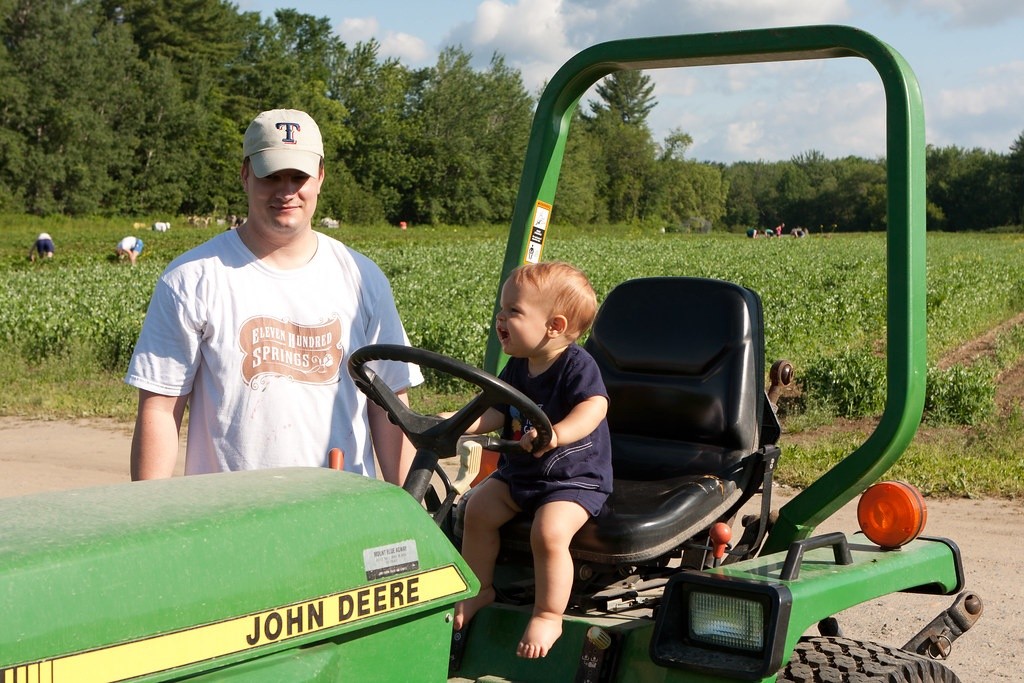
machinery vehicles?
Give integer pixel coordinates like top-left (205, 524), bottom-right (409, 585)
top-left (0, 22), bottom-right (983, 683)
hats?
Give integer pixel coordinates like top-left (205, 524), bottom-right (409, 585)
top-left (241, 107), bottom-right (325, 179)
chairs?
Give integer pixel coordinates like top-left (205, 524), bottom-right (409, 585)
top-left (453, 277), bottom-right (782, 564)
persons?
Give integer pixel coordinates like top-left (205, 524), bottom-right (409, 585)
top-left (116, 236), bottom-right (143, 266)
top-left (153, 221), bottom-right (170, 232)
top-left (762, 229), bottom-right (773, 238)
top-left (124, 109), bottom-right (424, 488)
top-left (776, 223), bottom-right (785, 236)
top-left (747, 227), bottom-right (760, 238)
top-left (400, 221), bottom-right (407, 230)
top-left (321, 216), bottom-right (339, 228)
top-left (30, 233), bottom-right (55, 261)
top-left (188, 214), bottom-right (247, 230)
top-left (791, 225), bottom-right (809, 238)
top-left (436, 262), bottom-right (612, 657)
